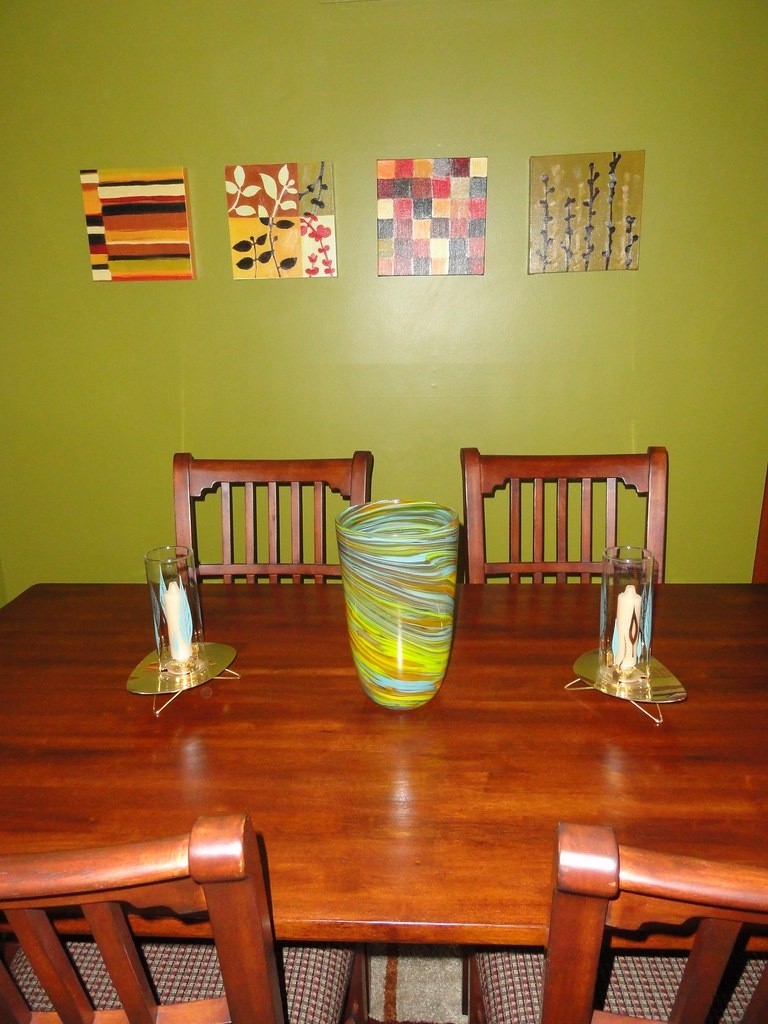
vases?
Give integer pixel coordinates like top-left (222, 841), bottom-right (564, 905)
top-left (336, 500), bottom-right (459, 711)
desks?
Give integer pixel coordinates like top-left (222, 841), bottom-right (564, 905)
top-left (1, 582), bottom-right (768, 956)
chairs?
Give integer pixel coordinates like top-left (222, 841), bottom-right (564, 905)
top-left (460, 447), bottom-right (670, 585)
top-left (462, 820), bottom-right (768, 1024)
top-left (1, 815), bottom-right (374, 1024)
top-left (173, 450), bottom-right (374, 585)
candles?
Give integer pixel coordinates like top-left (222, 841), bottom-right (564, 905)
top-left (162, 581), bottom-right (193, 661)
top-left (615, 585), bottom-right (642, 672)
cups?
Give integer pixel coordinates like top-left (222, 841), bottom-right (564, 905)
top-left (597, 546), bottom-right (654, 690)
top-left (145, 545), bottom-right (208, 679)
top-left (335, 499), bottom-right (460, 710)
